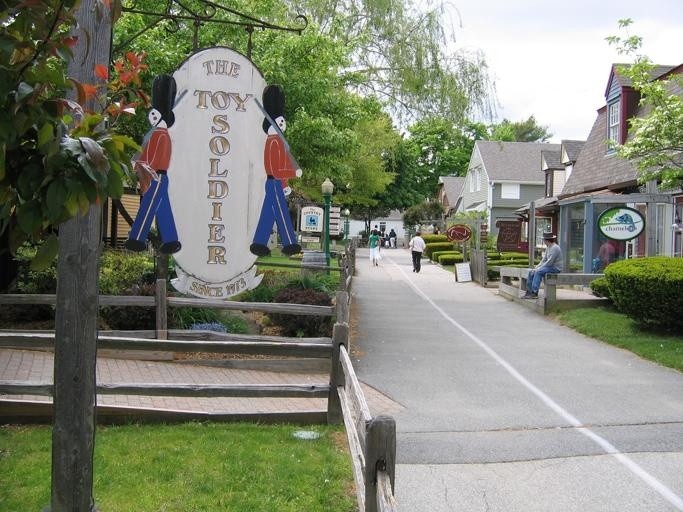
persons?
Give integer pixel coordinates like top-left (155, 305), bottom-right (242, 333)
top-left (369, 225), bottom-right (396, 248)
top-left (368, 231), bottom-right (381, 266)
top-left (521, 233), bottom-right (563, 299)
top-left (409, 232), bottom-right (426, 273)
top-left (597, 240), bottom-right (616, 258)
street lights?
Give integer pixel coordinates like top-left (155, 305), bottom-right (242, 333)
top-left (322, 177), bottom-right (334, 275)
top-left (345, 208), bottom-right (350, 251)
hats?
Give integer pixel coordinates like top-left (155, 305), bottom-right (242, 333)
top-left (541, 232), bottom-right (556, 239)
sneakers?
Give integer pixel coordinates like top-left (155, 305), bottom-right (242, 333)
top-left (521, 291), bottom-right (538, 299)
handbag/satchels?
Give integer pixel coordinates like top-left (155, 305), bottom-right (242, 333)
top-left (374, 235), bottom-right (378, 245)
